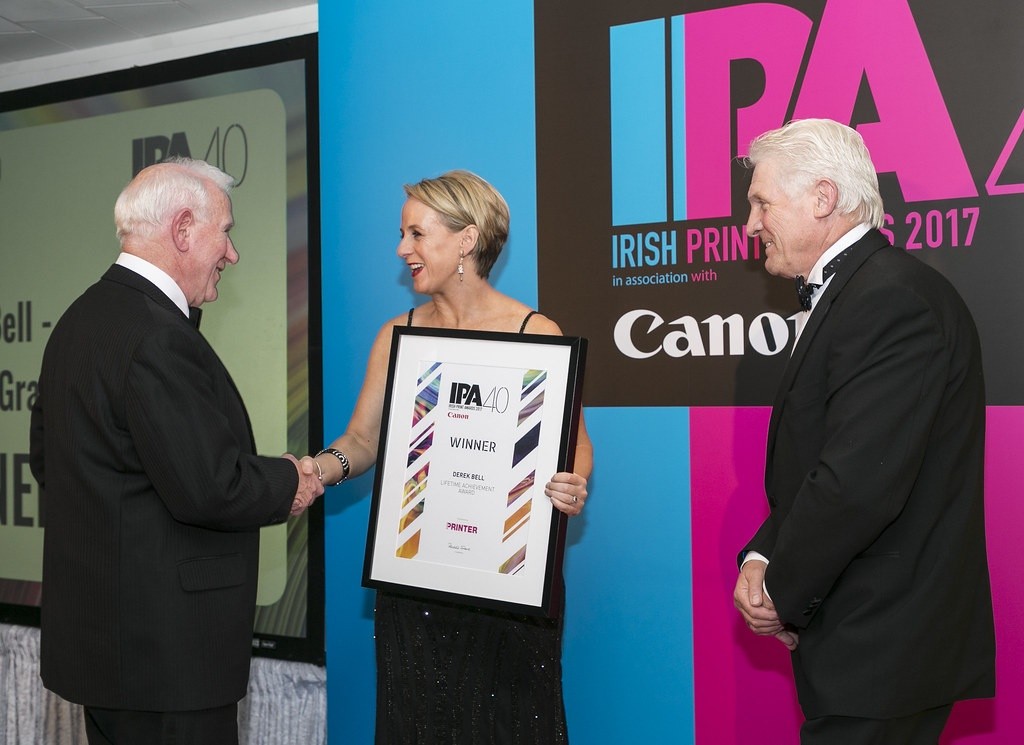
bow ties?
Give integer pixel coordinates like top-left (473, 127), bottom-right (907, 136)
top-left (794, 241), bottom-right (862, 311)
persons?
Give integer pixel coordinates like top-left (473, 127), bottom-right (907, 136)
top-left (28, 157), bottom-right (325, 745)
top-left (733, 118), bottom-right (997, 745)
top-left (294, 170), bottom-right (595, 745)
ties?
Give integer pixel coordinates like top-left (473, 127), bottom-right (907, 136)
top-left (188, 306), bottom-right (203, 331)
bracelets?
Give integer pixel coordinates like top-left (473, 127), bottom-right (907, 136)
top-left (303, 455), bottom-right (323, 480)
top-left (316, 448), bottom-right (350, 486)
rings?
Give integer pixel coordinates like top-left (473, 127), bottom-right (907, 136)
top-left (571, 496), bottom-right (578, 503)
top-left (747, 622), bottom-right (751, 626)
